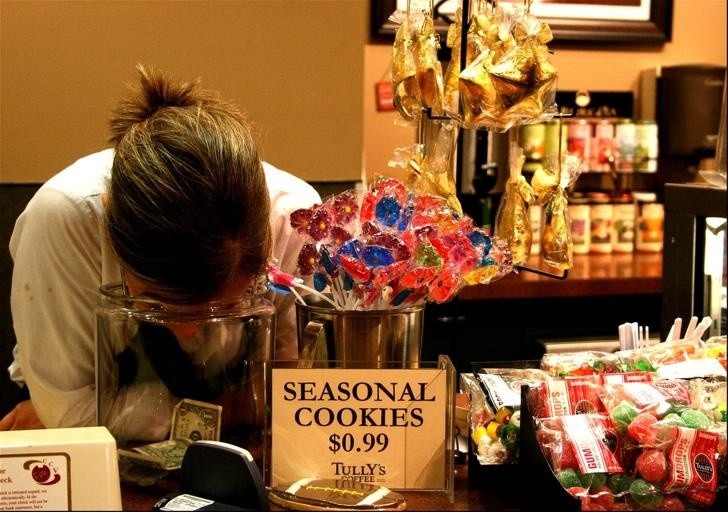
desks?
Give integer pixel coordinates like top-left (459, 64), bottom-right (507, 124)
top-left (0, 392), bottom-right (486, 512)
top-left (427, 254), bottom-right (669, 302)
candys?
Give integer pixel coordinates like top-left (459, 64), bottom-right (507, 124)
top-left (469, 334), bottom-right (727, 512)
top-left (266, 174), bottom-right (514, 310)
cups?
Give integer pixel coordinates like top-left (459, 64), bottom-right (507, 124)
top-left (294, 293), bottom-right (426, 369)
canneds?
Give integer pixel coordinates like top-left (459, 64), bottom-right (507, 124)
top-left (526, 116), bottom-right (665, 255)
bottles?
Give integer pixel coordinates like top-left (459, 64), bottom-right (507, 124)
top-left (92, 284), bottom-right (274, 495)
top-left (568, 190), bottom-right (663, 255)
top-left (525, 162), bottom-right (659, 175)
top-left (523, 118), bottom-right (660, 161)
top-left (569, 256), bottom-right (661, 278)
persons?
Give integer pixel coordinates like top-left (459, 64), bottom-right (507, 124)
top-left (8, 62), bottom-right (325, 446)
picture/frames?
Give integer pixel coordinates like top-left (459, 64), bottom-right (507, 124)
top-left (372, 0), bottom-right (677, 49)
